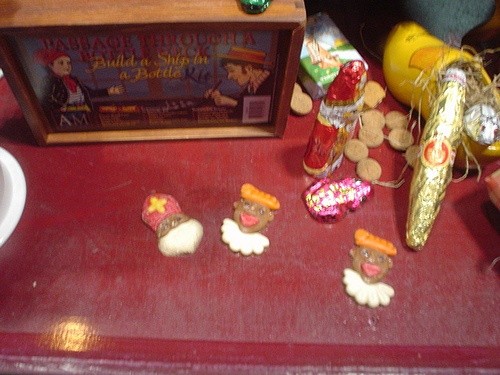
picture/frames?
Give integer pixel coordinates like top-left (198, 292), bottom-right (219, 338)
top-left (1, 0), bottom-right (307, 147)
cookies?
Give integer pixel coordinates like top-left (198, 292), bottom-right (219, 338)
top-left (290, 79), bottom-right (423, 182)
